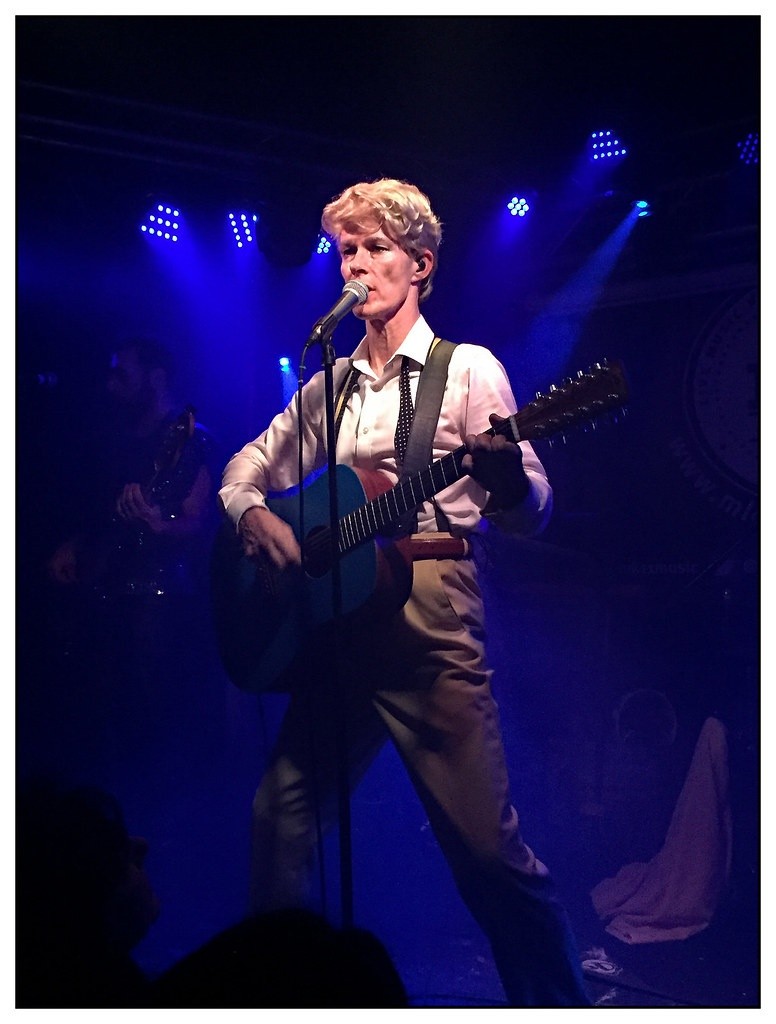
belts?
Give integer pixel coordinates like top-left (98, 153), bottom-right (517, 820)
top-left (410, 535), bottom-right (477, 562)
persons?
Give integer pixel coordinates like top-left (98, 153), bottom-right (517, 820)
top-left (51, 339), bottom-right (211, 581)
top-left (217, 179), bottom-right (590, 1009)
top-left (16, 777), bottom-right (408, 1007)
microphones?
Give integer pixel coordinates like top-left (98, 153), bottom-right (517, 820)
top-left (305, 279), bottom-right (369, 347)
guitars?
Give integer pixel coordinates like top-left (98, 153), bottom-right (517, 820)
top-left (204, 348), bottom-right (637, 702)
